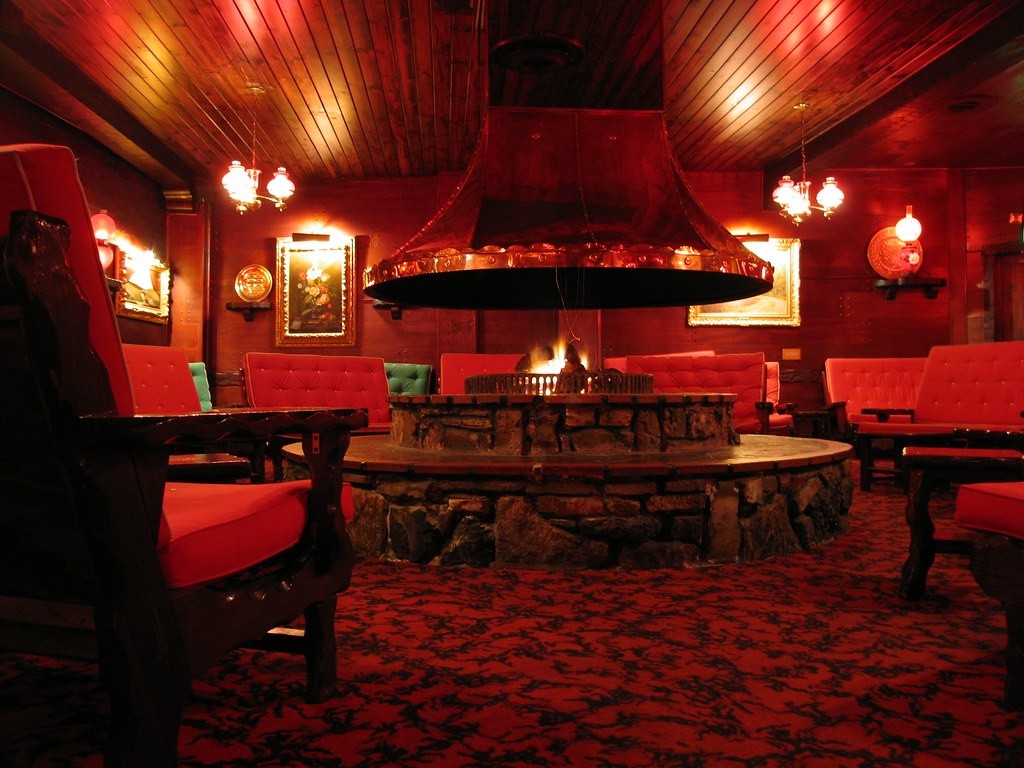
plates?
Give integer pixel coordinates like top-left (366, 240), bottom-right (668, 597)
top-left (868, 226), bottom-right (923, 280)
top-left (234, 263), bottom-right (273, 303)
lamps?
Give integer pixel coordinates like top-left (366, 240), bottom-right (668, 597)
top-left (92, 210), bottom-right (116, 269)
top-left (220, 85), bottom-right (296, 214)
top-left (772, 104), bottom-right (844, 225)
top-left (896, 206), bottom-right (921, 272)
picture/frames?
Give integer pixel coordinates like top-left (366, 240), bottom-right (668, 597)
top-left (272, 234), bottom-right (358, 346)
top-left (686, 235), bottom-right (802, 328)
top-left (115, 256), bottom-right (171, 325)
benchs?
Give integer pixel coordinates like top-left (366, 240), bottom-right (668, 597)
top-left (1, 145), bottom-right (1024, 768)
